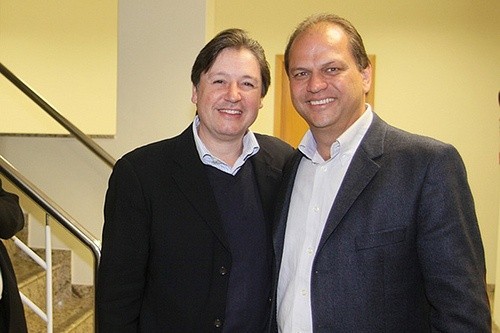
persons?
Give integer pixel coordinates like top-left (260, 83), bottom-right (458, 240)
top-left (255, 9), bottom-right (494, 333)
top-left (95, 29), bottom-right (301, 333)
top-left (0, 176), bottom-right (31, 333)
